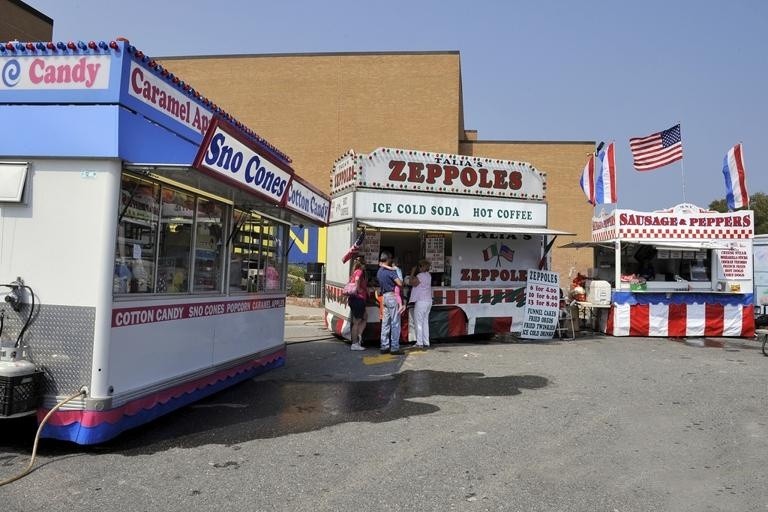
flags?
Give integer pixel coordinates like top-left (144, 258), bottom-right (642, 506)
top-left (595, 141), bottom-right (618, 204)
top-left (627, 121), bottom-right (683, 173)
top-left (580, 155), bottom-right (596, 207)
top-left (721, 143), bottom-right (750, 210)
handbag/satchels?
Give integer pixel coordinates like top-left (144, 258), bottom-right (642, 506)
top-left (342, 283), bottom-right (357, 296)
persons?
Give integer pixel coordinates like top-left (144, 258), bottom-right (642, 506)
top-left (378, 256), bottom-right (406, 315)
top-left (409, 259), bottom-right (434, 349)
top-left (375, 287), bottom-right (383, 343)
top-left (347, 256), bottom-right (370, 351)
top-left (376, 252), bottom-right (406, 355)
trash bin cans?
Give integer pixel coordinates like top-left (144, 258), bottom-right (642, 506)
top-left (304, 262), bottom-right (326, 299)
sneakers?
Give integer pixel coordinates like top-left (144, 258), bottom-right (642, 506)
top-left (391, 350), bottom-right (405, 355)
top-left (358, 334), bottom-right (362, 344)
top-left (381, 349), bottom-right (388, 353)
top-left (350, 343), bottom-right (367, 350)
